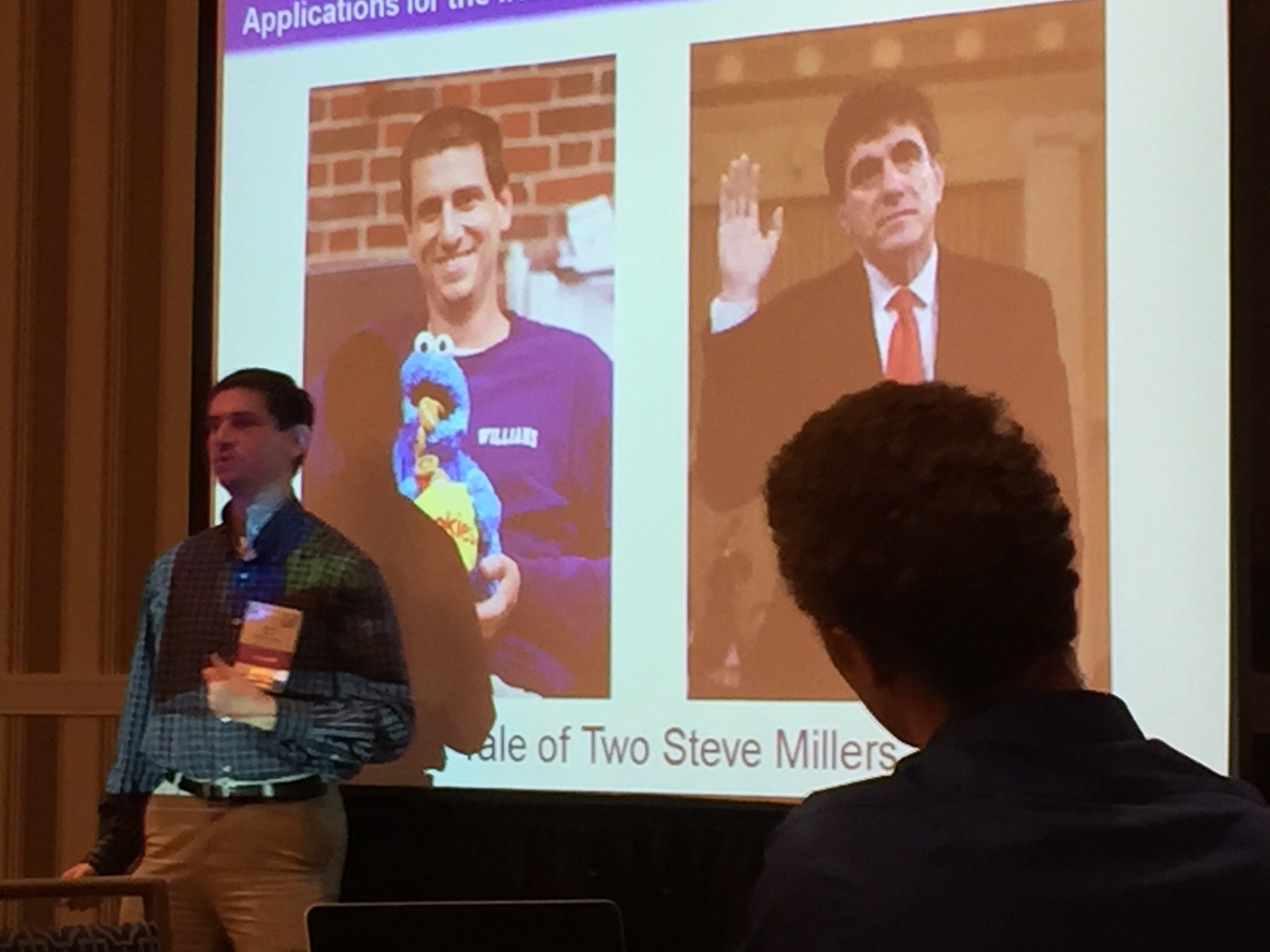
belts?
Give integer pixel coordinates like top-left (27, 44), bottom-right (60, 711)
top-left (164, 768), bottom-right (329, 808)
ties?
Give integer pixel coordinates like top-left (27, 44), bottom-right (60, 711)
top-left (885, 288), bottom-right (926, 385)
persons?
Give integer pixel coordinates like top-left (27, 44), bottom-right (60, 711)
top-left (56, 369), bottom-right (415, 952)
top-left (701, 83), bottom-right (1077, 685)
top-left (749, 377), bottom-right (1270, 952)
top-left (305, 107), bottom-right (611, 703)
